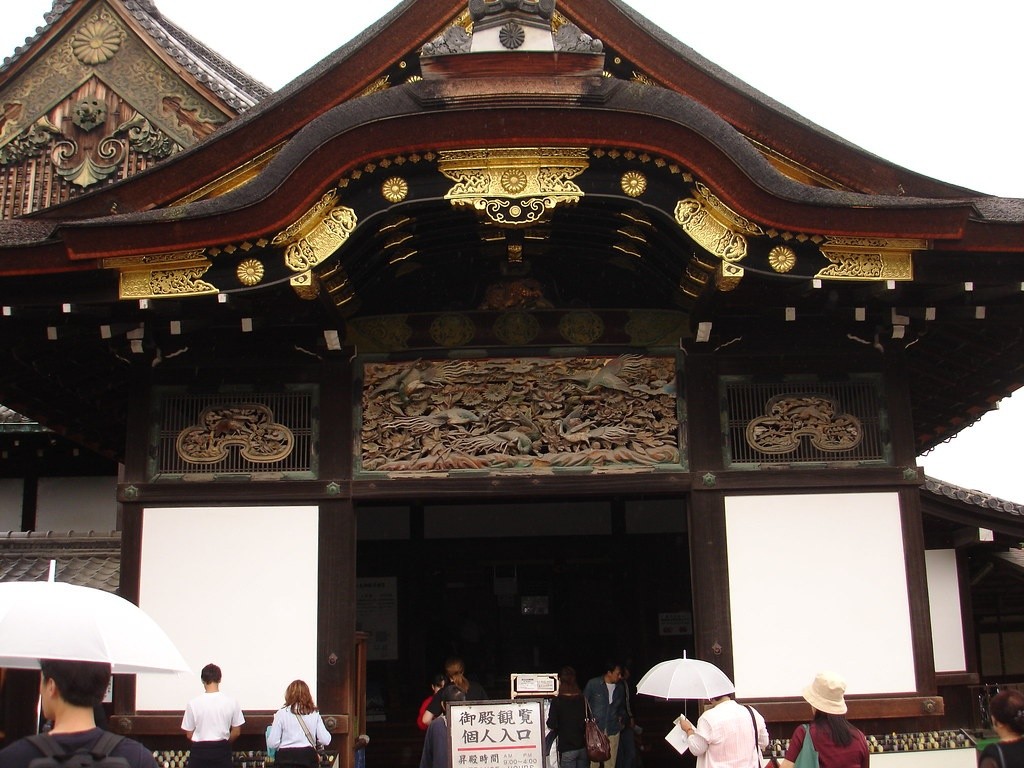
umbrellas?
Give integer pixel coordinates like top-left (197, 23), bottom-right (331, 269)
top-left (635, 651), bottom-right (736, 731)
top-left (0, 560), bottom-right (186, 737)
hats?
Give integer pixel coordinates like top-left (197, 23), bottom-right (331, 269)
top-left (801, 673), bottom-right (848, 714)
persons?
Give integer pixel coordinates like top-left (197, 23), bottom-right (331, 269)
top-left (417, 671), bottom-right (447, 730)
top-left (546, 667), bottom-right (594, 768)
top-left (181, 663), bottom-right (245, 768)
top-left (980, 689), bottom-right (1024, 768)
top-left (680, 696), bottom-right (770, 768)
top-left (776, 674), bottom-right (870, 768)
top-left (420, 687), bottom-right (466, 768)
top-left (425, 657), bottom-right (488, 726)
top-left (267, 680), bottom-right (332, 768)
top-left (0, 658), bottom-right (158, 768)
top-left (583, 664), bottom-right (628, 768)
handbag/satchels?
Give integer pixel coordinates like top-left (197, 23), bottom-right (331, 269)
top-left (793, 724), bottom-right (820, 768)
top-left (584, 694), bottom-right (612, 762)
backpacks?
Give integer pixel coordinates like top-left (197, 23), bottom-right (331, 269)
top-left (25, 730), bottom-right (131, 768)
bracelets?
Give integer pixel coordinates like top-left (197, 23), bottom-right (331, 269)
top-left (686, 728), bottom-right (693, 733)
